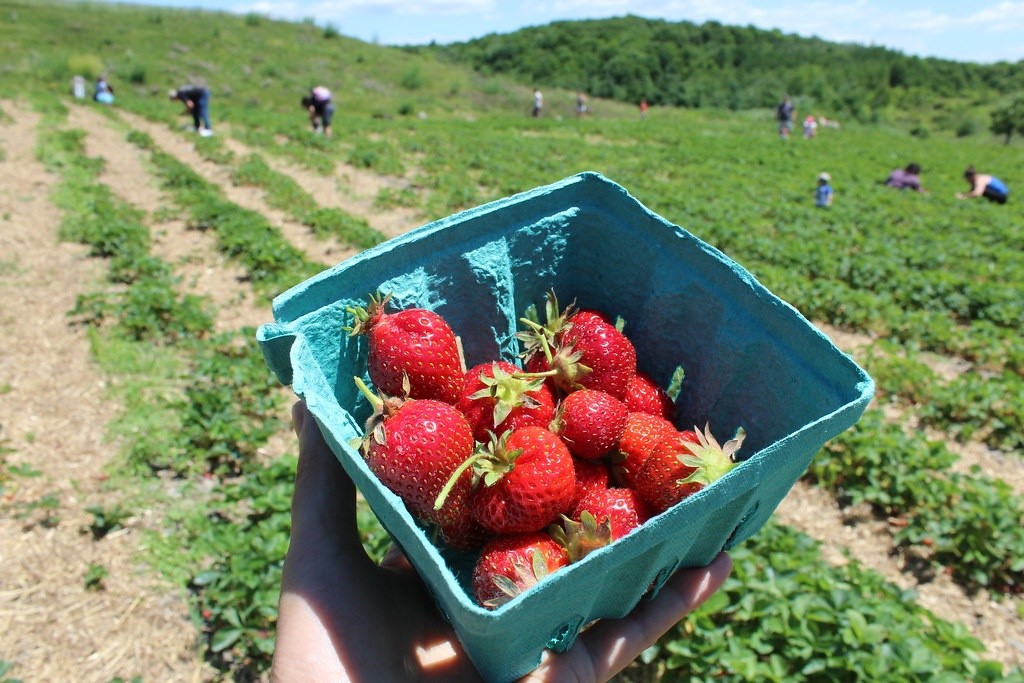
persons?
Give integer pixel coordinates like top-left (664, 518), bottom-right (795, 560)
top-left (301, 85), bottom-right (337, 138)
top-left (531, 88), bottom-right (545, 118)
top-left (955, 165), bottom-right (1009, 206)
top-left (266, 399), bottom-right (733, 683)
top-left (639, 97), bottom-right (647, 121)
top-left (813, 172), bottom-right (834, 208)
top-left (574, 91), bottom-right (590, 120)
top-left (776, 96), bottom-right (839, 137)
top-left (168, 83), bottom-right (217, 136)
top-left (883, 162), bottom-right (931, 196)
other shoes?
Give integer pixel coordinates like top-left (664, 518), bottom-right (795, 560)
top-left (187, 125), bottom-right (214, 137)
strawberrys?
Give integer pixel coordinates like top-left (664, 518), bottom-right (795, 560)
top-left (344, 286), bottom-right (746, 604)
top-left (202, 610), bottom-right (210, 618)
top-left (895, 517), bottom-right (1022, 594)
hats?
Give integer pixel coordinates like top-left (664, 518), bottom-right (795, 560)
top-left (818, 173), bottom-right (831, 181)
